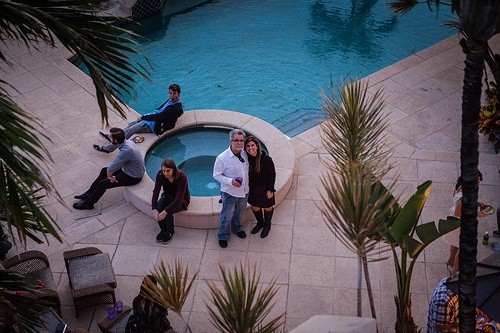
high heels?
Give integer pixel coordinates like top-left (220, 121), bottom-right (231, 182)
top-left (445, 262), bottom-right (452, 269)
top-left (449, 269), bottom-right (458, 278)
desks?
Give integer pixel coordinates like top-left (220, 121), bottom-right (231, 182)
top-left (11, 307), bottom-right (70, 333)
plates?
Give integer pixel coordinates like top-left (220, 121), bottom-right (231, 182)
top-left (489, 242), bottom-right (500, 252)
top-left (480, 204), bottom-right (496, 216)
top-left (133, 136), bottom-right (144, 142)
top-left (473, 315), bottom-right (496, 333)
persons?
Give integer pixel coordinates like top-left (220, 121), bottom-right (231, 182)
top-left (492, 169), bottom-right (500, 237)
top-left (244, 134), bottom-right (276, 238)
top-left (446, 169), bottom-right (483, 277)
top-left (132, 274), bottom-right (177, 333)
top-left (93, 84), bottom-right (184, 154)
top-left (71, 127), bottom-right (145, 209)
top-left (150, 158), bottom-right (190, 243)
top-left (427, 273), bottom-right (500, 333)
top-left (211, 129), bottom-right (250, 248)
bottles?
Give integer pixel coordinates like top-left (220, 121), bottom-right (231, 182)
top-left (482, 231), bottom-right (489, 245)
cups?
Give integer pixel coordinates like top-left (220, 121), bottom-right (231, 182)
top-left (116, 301), bottom-right (123, 312)
top-left (107, 307), bottom-right (116, 320)
top-left (235, 177), bottom-right (243, 186)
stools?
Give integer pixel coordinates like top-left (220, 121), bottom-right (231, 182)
top-left (63, 247), bottom-right (117, 317)
top-left (96, 304), bottom-right (174, 333)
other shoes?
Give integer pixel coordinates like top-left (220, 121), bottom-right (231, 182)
top-left (92, 144), bottom-right (110, 154)
top-left (73, 202), bottom-right (94, 210)
top-left (236, 230), bottom-right (246, 238)
top-left (74, 195), bottom-right (85, 200)
top-left (156, 231), bottom-right (166, 241)
top-left (99, 131), bottom-right (111, 142)
top-left (162, 231), bottom-right (174, 243)
top-left (219, 239), bottom-right (227, 248)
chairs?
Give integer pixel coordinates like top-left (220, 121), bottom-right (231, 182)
top-left (0, 250), bottom-right (62, 318)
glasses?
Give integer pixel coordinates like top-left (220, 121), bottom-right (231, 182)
top-left (232, 139), bottom-right (243, 143)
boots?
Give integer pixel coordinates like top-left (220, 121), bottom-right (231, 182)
top-left (260, 208), bottom-right (274, 238)
top-left (250, 207), bottom-right (263, 234)
